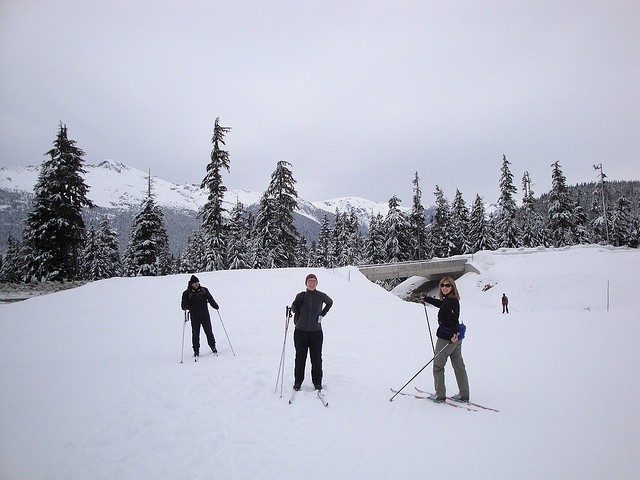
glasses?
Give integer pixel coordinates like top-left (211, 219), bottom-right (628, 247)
top-left (307, 280), bottom-right (317, 283)
top-left (440, 283), bottom-right (452, 287)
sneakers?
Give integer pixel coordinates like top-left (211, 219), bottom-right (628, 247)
top-left (427, 394), bottom-right (446, 400)
top-left (314, 383), bottom-right (322, 390)
top-left (194, 351), bottom-right (199, 357)
top-left (211, 346), bottom-right (217, 353)
top-left (452, 394), bottom-right (469, 400)
top-left (293, 383), bottom-right (301, 389)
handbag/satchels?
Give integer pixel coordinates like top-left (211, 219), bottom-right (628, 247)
top-left (438, 322), bottom-right (466, 341)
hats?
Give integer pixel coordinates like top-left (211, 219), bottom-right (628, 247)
top-left (190, 275), bottom-right (199, 285)
top-left (305, 274), bottom-right (318, 286)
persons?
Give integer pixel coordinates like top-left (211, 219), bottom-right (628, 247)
top-left (501, 294), bottom-right (510, 313)
top-left (420, 278), bottom-right (474, 403)
top-left (290, 275), bottom-right (334, 393)
top-left (181, 275), bottom-right (220, 357)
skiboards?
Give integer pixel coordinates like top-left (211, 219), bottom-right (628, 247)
top-left (289, 390), bottom-right (329, 407)
top-left (390, 387), bottom-right (500, 413)
top-left (195, 353), bottom-right (219, 361)
